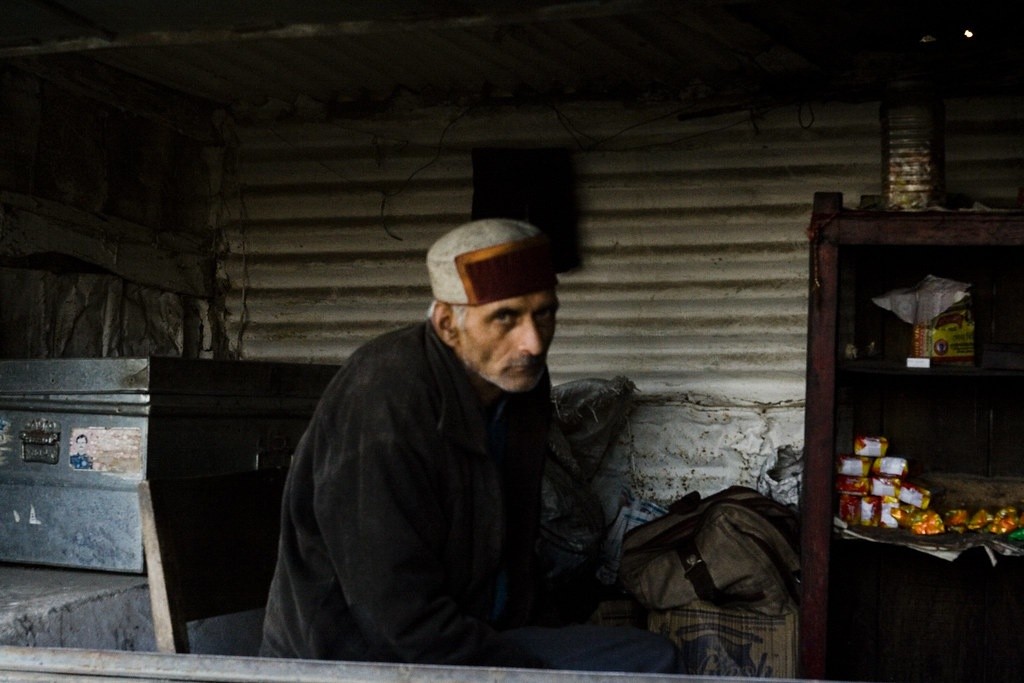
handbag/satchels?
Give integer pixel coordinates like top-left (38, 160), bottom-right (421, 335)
top-left (614, 484), bottom-right (806, 617)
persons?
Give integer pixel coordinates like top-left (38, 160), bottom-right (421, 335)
top-left (259, 218), bottom-right (683, 672)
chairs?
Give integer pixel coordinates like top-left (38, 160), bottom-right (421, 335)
top-left (138, 464), bottom-right (285, 658)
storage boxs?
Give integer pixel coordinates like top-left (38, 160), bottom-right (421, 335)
top-left (646, 602), bottom-right (800, 679)
top-left (0, 357), bottom-right (344, 574)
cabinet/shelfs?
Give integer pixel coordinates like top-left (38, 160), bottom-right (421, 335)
top-left (802, 192), bottom-right (1024, 683)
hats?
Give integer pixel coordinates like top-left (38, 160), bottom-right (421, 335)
top-left (425, 218), bottom-right (558, 305)
